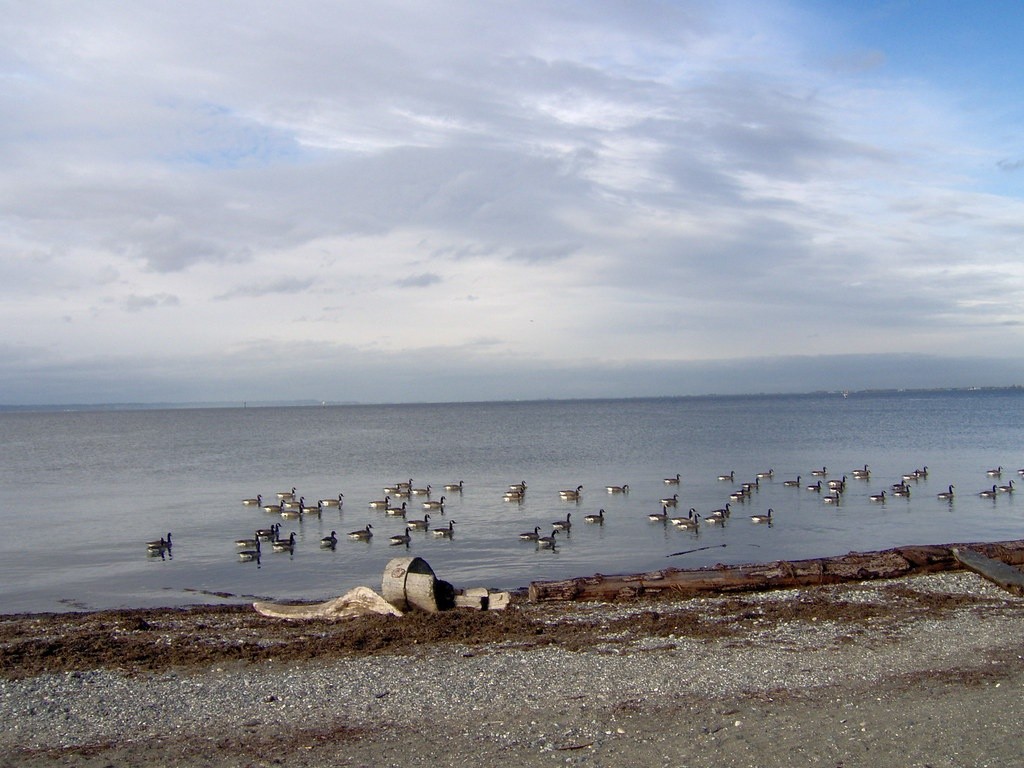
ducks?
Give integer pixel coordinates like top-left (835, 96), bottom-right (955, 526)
top-left (501, 463), bottom-right (1024, 549)
top-left (145, 532), bottom-right (173, 553)
top-left (234, 477), bottom-right (465, 561)
